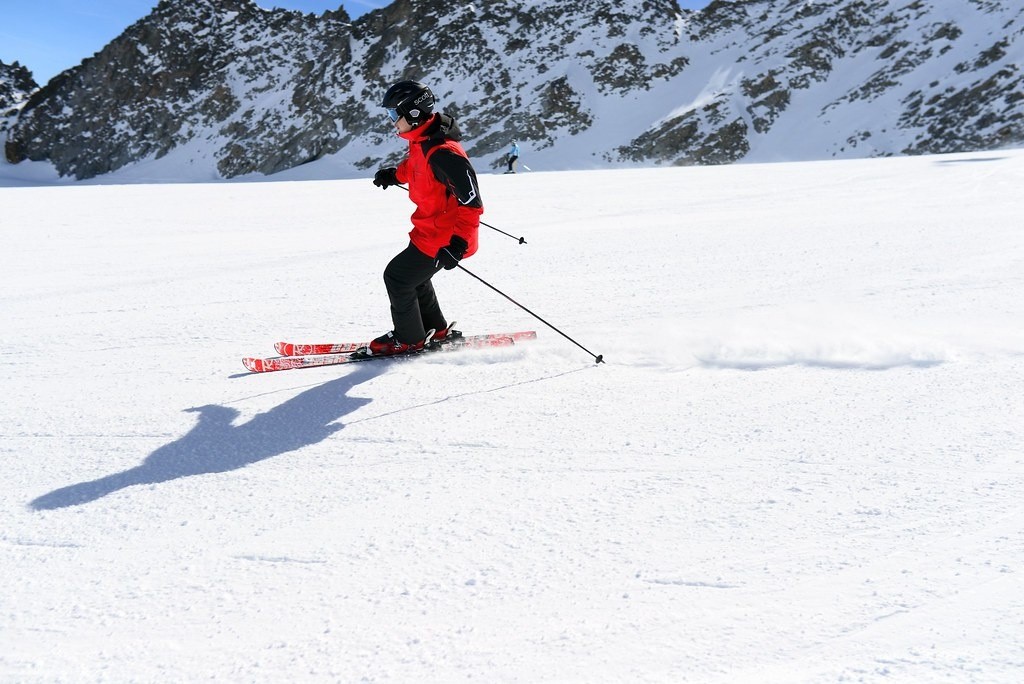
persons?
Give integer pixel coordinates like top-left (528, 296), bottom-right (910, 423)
top-left (370, 81), bottom-right (484, 354)
top-left (508, 139), bottom-right (519, 172)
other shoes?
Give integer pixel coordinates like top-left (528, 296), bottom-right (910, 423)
top-left (369, 321), bottom-right (460, 355)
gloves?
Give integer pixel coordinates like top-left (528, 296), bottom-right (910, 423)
top-left (373, 167), bottom-right (406, 190)
top-left (433, 234), bottom-right (469, 270)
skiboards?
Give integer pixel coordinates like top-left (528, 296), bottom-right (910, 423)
top-left (242, 331), bottom-right (537, 372)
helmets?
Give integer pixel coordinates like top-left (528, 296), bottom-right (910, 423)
top-left (381, 80), bottom-right (436, 131)
